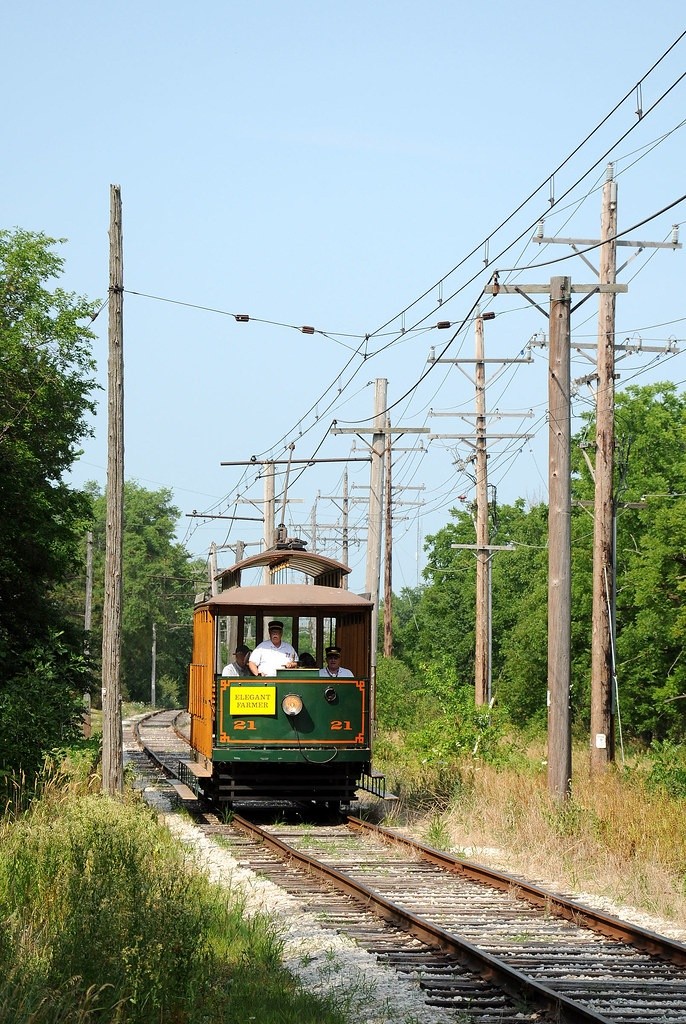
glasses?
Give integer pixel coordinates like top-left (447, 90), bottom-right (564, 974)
top-left (329, 657), bottom-right (338, 660)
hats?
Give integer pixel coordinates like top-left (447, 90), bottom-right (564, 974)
top-left (326, 647), bottom-right (342, 657)
top-left (232, 645), bottom-right (252, 655)
top-left (268, 621), bottom-right (284, 630)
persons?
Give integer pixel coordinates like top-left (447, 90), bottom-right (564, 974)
top-left (249, 621), bottom-right (299, 676)
top-left (299, 653), bottom-right (318, 668)
top-left (319, 647), bottom-right (354, 677)
top-left (222, 645), bottom-right (253, 676)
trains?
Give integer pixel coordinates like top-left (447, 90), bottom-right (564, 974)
top-left (167, 442), bottom-right (400, 814)
